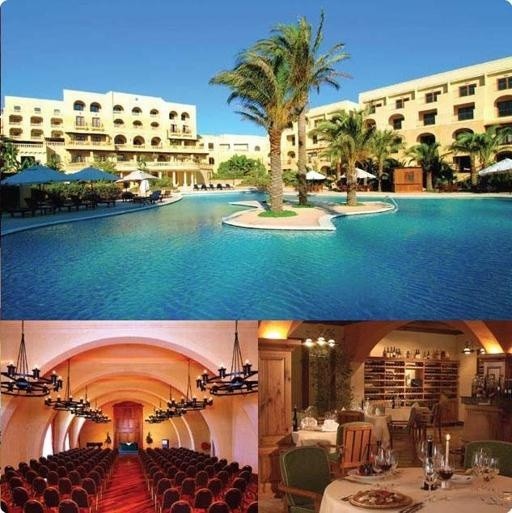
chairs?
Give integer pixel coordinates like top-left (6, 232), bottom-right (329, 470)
top-left (278, 422), bottom-right (511, 511)
top-left (0, 448), bottom-right (119, 512)
top-left (137, 447), bottom-right (258, 513)
top-left (1, 187), bottom-right (163, 216)
top-left (301, 410), bottom-right (363, 431)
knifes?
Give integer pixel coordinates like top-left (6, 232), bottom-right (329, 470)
top-left (398, 493), bottom-right (435, 513)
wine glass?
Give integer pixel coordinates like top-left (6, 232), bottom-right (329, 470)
top-left (371, 439), bottom-right (499, 504)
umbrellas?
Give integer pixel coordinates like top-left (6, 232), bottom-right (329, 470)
top-left (339, 167), bottom-right (377, 179)
top-left (115, 169), bottom-right (161, 198)
top-left (139, 179), bottom-right (149, 194)
top-left (0, 162), bottom-right (71, 215)
top-left (66, 164), bottom-right (120, 199)
top-left (305, 171), bottom-right (326, 188)
top-left (479, 158), bottom-right (512, 176)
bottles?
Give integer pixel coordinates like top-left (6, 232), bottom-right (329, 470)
top-left (383, 346), bottom-right (430, 359)
top-left (496, 375), bottom-right (512, 401)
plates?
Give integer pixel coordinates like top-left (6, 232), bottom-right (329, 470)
top-left (349, 489), bottom-right (413, 508)
top-left (347, 468), bottom-right (386, 483)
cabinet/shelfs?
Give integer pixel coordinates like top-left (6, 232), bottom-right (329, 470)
top-left (363, 356), bottom-right (459, 421)
top-left (258, 339), bottom-right (302, 493)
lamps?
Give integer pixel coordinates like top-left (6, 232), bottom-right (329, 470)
top-left (144, 321), bottom-right (258, 423)
top-left (1, 321), bottom-right (112, 423)
